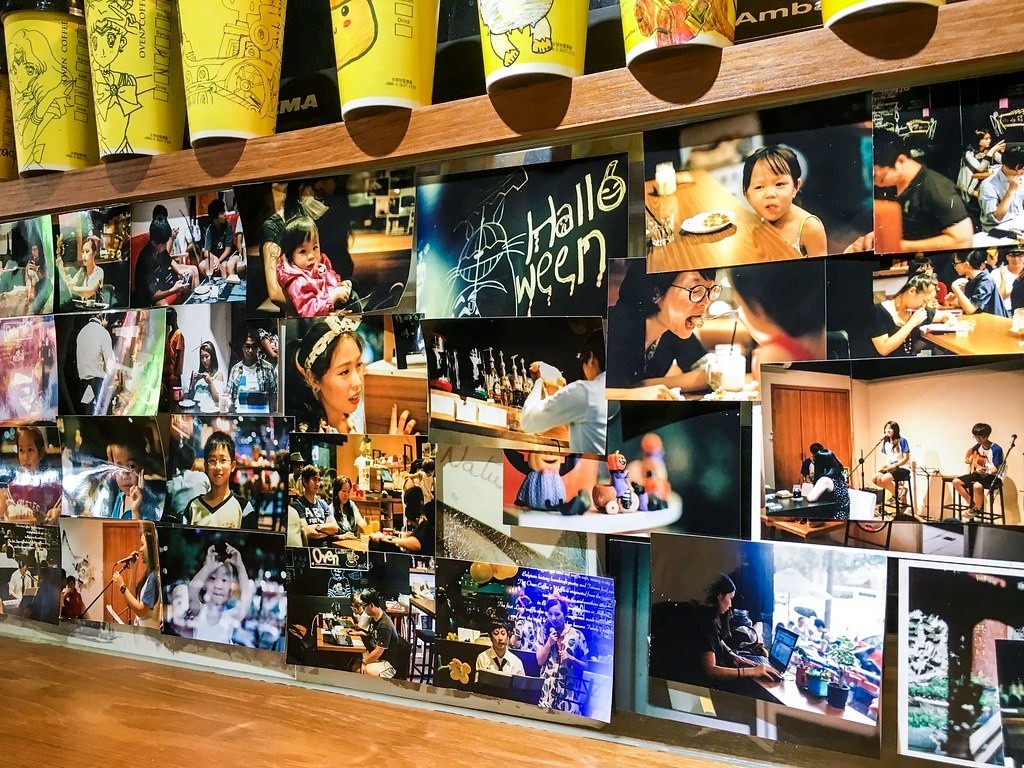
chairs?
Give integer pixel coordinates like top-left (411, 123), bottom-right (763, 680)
top-left (393, 636), bottom-right (411, 681)
top-left (186, 212), bottom-right (247, 280)
top-left (843, 488), bottom-right (893, 550)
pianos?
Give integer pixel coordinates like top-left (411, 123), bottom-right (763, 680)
top-left (2, 600), bottom-right (21, 615)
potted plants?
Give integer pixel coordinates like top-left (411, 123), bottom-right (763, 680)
top-left (826, 637), bottom-right (857, 709)
top-left (904, 676), bottom-right (952, 749)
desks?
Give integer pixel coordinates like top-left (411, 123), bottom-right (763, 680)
top-left (316, 598), bottom-right (436, 675)
top-left (750, 670), bottom-right (877, 726)
top-left (919, 310), bottom-right (1024, 355)
top-left (644, 168), bottom-right (805, 274)
top-left (972, 170), bottom-right (989, 179)
top-left (230, 466), bottom-right (283, 529)
top-left (168, 392), bottom-right (277, 415)
top-left (504, 477), bottom-right (683, 536)
top-left (350, 497), bottom-right (403, 536)
top-left (331, 538), bottom-right (371, 552)
top-left (761, 487), bottom-right (849, 547)
top-left (183, 276), bottom-right (248, 305)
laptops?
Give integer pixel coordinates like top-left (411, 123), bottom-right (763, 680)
top-left (325, 619), bottom-right (354, 647)
top-left (739, 625), bottom-right (799, 669)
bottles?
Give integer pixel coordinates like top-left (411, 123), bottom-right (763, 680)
top-left (714, 345), bottom-right (745, 390)
top-left (656, 162), bottom-right (677, 195)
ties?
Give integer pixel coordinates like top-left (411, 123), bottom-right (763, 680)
top-left (493, 656), bottom-right (507, 672)
top-left (21, 576), bottom-right (25, 596)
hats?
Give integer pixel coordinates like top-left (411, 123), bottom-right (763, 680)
top-left (290, 451), bottom-right (305, 463)
top-left (1007, 246), bottom-right (1024, 253)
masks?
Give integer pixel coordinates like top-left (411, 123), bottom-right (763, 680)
top-left (299, 186), bottom-right (329, 220)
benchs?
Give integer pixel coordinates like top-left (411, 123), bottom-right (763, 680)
top-left (132, 232), bottom-right (190, 306)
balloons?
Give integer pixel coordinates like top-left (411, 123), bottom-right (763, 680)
top-left (470, 562), bottom-right (518, 583)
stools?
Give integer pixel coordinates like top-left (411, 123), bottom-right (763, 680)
top-left (940, 476), bottom-right (1005, 525)
top-left (881, 476), bottom-right (914, 522)
top-left (410, 629), bottom-right (436, 684)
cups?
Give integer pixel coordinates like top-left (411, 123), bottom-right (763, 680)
top-left (0, 0), bottom-right (946, 181)
top-left (792, 484), bottom-right (801, 498)
top-left (171, 386), bottom-right (184, 400)
top-left (647, 208), bottom-right (675, 246)
top-left (218, 394), bottom-right (230, 414)
top-left (954, 316), bottom-right (970, 337)
top-left (1013, 308), bottom-right (1024, 343)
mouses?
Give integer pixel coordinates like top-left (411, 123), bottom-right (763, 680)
top-left (347, 630), bottom-right (352, 634)
top-left (760, 672), bottom-right (782, 683)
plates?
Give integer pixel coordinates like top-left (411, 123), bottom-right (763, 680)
top-left (193, 286), bottom-right (209, 294)
top-left (178, 399), bottom-right (197, 407)
top-left (681, 209), bottom-right (735, 233)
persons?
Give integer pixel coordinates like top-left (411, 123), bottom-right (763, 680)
top-left (258, 175), bottom-right (364, 318)
top-left (25, 235), bottom-right (53, 313)
top-left (287, 452), bottom-right (367, 547)
top-left (76, 313), bottom-right (118, 414)
top-left (743, 146), bottom-right (828, 257)
top-left (965, 130), bottom-right (1024, 232)
top-left (135, 204), bottom-right (199, 306)
top-left (285, 317), bottom-right (421, 435)
top-left (865, 244), bottom-right (1024, 358)
top-left (161, 306), bottom-right (278, 413)
top-left (875, 127), bottom-right (973, 252)
top-left (521, 328), bottom-right (608, 455)
top-left (43, 531), bottom-right (400, 679)
top-left (504, 433), bottom-right (670, 515)
top-left (0, 425), bottom-right (62, 524)
top-left (605, 256), bottom-right (826, 400)
top-left (199, 200), bottom-right (248, 279)
top-left (0, 527), bottom-right (49, 616)
top-left (354, 442), bottom-right (435, 556)
top-left (474, 574), bottom-right (830, 716)
top-left (111, 423), bottom-right (162, 521)
top-left (799, 419), bottom-right (1004, 519)
top-left (163, 432), bottom-right (258, 529)
top-left (63, 230), bottom-right (103, 302)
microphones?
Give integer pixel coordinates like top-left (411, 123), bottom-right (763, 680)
top-left (1010, 435), bottom-right (1017, 446)
top-left (550, 627), bottom-right (558, 646)
top-left (115, 555), bottom-right (136, 565)
top-left (881, 434), bottom-right (888, 440)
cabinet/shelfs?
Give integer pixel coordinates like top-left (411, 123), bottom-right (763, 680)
top-left (7, 525), bottom-right (49, 550)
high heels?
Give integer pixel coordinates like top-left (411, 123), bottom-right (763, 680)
top-left (963, 503), bottom-right (984, 519)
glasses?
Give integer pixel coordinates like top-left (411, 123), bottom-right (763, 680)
top-left (310, 183), bottom-right (334, 206)
top-left (362, 602), bottom-right (373, 608)
top-left (670, 285), bottom-right (723, 303)
top-left (952, 259), bottom-right (967, 266)
top-left (242, 344), bottom-right (260, 350)
top-left (206, 458), bottom-right (232, 466)
top-left (350, 604), bottom-right (362, 609)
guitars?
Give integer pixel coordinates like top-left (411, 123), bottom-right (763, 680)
top-left (969, 450), bottom-right (987, 475)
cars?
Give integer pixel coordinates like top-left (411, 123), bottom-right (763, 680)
top-left (774, 594), bottom-right (788, 605)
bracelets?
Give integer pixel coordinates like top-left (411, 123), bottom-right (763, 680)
top-left (737, 667), bottom-right (745, 677)
top-left (727, 649), bottom-right (732, 653)
